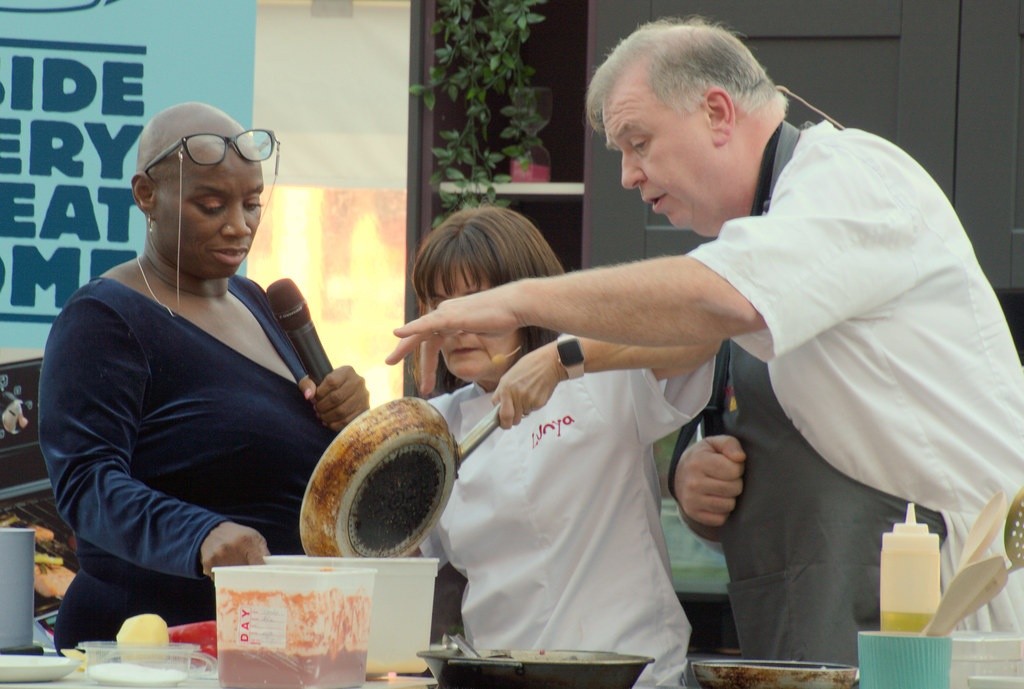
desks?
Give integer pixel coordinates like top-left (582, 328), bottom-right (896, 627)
top-left (0, 673), bottom-right (436, 689)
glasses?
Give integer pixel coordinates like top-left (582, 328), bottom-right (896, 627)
top-left (143, 128), bottom-right (276, 174)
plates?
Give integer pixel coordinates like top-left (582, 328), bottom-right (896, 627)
top-left (0, 655), bottom-right (81, 683)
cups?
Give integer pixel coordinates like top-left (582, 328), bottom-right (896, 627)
top-left (857, 630), bottom-right (953, 689)
top-left (0, 527), bottom-right (36, 654)
top-left (946, 632), bottom-right (1024, 689)
top-left (79, 641), bottom-right (218, 689)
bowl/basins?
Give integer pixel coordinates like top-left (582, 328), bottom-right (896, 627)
top-left (262, 555), bottom-right (440, 675)
top-left (690, 659), bottom-right (857, 689)
top-left (416, 649), bottom-right (656, 689)
top-left (211, 565), bottom-right (377, 689)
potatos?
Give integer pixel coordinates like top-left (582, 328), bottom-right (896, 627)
top-left (117, 613), bottom-right (169, 664)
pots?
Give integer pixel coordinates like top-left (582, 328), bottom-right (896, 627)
top-left (299, 396), bottom-right (501, 558)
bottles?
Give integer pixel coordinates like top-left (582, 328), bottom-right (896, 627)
top-left (879, 503), bottom-right (941, 632)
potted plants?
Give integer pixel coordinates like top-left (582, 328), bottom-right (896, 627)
top-left (409, 0), bottom-right (550, 228)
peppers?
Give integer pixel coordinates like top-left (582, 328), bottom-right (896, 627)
top-left (167, 620), bottom-right (216, 666)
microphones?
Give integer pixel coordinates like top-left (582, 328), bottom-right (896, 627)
top-left (267, 278), bottom-right (334, 389)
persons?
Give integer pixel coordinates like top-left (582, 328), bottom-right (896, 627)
top-left (386, 14), bottom-right (1024, 668)
top-left (35, 103), bottom-right (370, 658)
top-left (407, 203), bottom-right (723, 689)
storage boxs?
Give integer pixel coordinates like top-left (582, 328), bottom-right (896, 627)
top-left (262, 555), bottom-right (440, 677)
top-left (211, 565), bottom-right (377, 689)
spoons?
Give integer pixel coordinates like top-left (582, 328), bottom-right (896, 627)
top-left (960, 489), bottom-right (1007, 565)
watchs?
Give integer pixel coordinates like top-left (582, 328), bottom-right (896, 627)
top-left (556, 331), bottom-right (588, 380)
